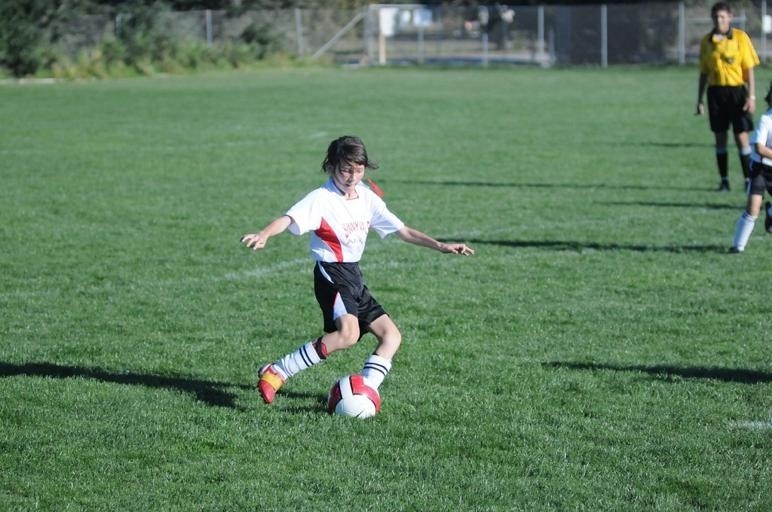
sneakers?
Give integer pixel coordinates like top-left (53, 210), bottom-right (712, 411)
top-left (720, 179), bottom-right (729, 192)
top-left (258, 363), bottom-right (286, 403)
top-left (729, 247), bottom-right (739, 253)
top-left (765, 201), bottom-right (772, 233)
top-left (744, 181), bottom-right (749, 191)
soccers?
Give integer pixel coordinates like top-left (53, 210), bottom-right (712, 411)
top-left (328, 375), bottom-right (380, 420)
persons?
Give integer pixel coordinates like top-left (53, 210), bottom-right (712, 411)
top-left (240, 136), bottom-right (475, 403)
top-left (695, 2), bottom-right (760, 194)
top-left (729, 82), bottom-right (772, 253)
